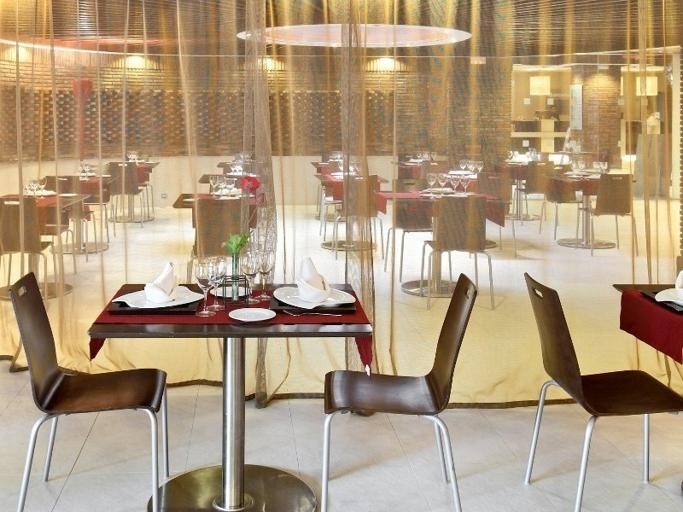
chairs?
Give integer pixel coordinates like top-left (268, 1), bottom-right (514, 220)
top-left (6, 269), bottom-right (171, 507)
top-left (522, 270), bottom-right (681, 507)
top-left (311, 147), bottom-right (511, 312)
top-left (314, 270), bottom-right (480, 507)
top-left (1, 151), bottom-right (161, 302)
top-left (504, 146), bottom-right (643, 259)
top-left (172, 150), bottom-right (268, 284)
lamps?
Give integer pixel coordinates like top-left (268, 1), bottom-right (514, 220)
top-left (528, 67), bottom-right (553, 98)
top-left (617, 64), bottom-right (666, 98)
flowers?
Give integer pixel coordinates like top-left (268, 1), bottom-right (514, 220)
top-left (220, 230), bottom-right (253, 257)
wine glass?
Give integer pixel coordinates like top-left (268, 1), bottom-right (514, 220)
top-left (193, 245), bottom-right (277, 319)
top-left (228, 148), bottom-right (254, 176)
top-left (569, 160), bottom-right (608, 175)
top-left (412, 150), bottom-right (485, 199)
top-left (126, 150), bottom-right (136, 160)
top-left (79, 160), bottom-right (90, 181)
top-left (21, 171), bottom-right (49, 201)
top-left (329, 150), bottom-right (365, 181)
top-left (208, 174), bottom-right (238, 200)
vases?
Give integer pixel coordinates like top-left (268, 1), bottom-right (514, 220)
top-left (227, 251), bottom-right (243, 302)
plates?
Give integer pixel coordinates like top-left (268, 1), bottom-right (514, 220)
top-left (111, 284), bottom-right (203, 309)
top-left (228, 306), bottom-right (277, 325)
top-left (271, 285), bottom-right (355, 310)
top-left (208, 285), bottom-right (252, 299)
